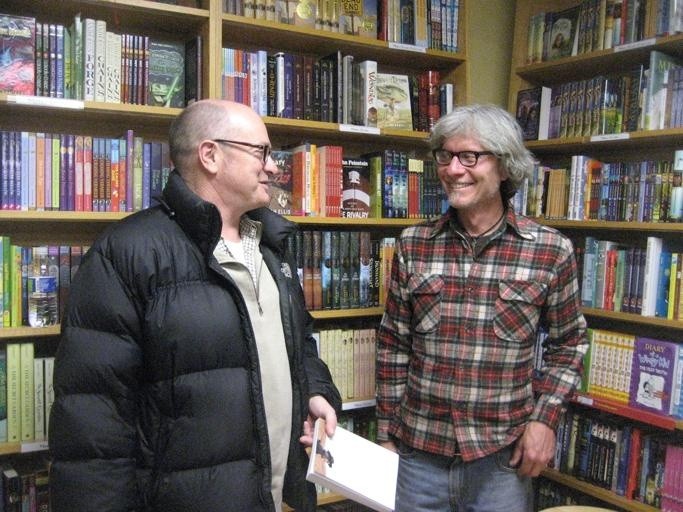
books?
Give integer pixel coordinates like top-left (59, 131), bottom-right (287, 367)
top-left (265, 140), bottom-right (450, 219)
top-left (516, 51), bottom-right (683, 140)
top-left (288, 230), bottom-right (400, 311)
top-left (314, 412), bottom-right (375, 492)
top-left (1, 236), bottom-right (90, 327)
top-left (526, 1), bottom-right (683, 64)
top-left (311, 323), bottom-right (378, 400)
top-left (531, 407), bottom-right (683, 512)
top-left (0, 129), bottom-right (175, 212)
top-left (1, 342), bottom-right (55, 511)
top-left (0, 14), bottom-right (201, 108)
top-left (305, 418), bottom-right (401, 512)
top-left (222, 0), bottom-right (460, 52)
top-left (222, 48), bottom-right (453, 132)
top-left (510, 150), bottom-right (682, 223)
top-left (532, 325), bottom-right (683, 416)
top-left (575, 235), bottom-right (683, 320)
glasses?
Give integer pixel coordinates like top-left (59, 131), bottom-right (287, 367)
top-left (432, 148), bottom-right (494, 168)
top-left (213, 139), bottom-right (273, 162)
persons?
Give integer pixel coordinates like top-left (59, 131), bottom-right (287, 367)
top-left (47, 98), bottom-right (342, 512)
top-left (372, 103), bottom-right (587, 512)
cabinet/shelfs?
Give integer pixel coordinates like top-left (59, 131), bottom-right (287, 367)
top-left (506, 4), bottom-right (683, 512)
top-left (0, 0), bottom-right (471, 509)
